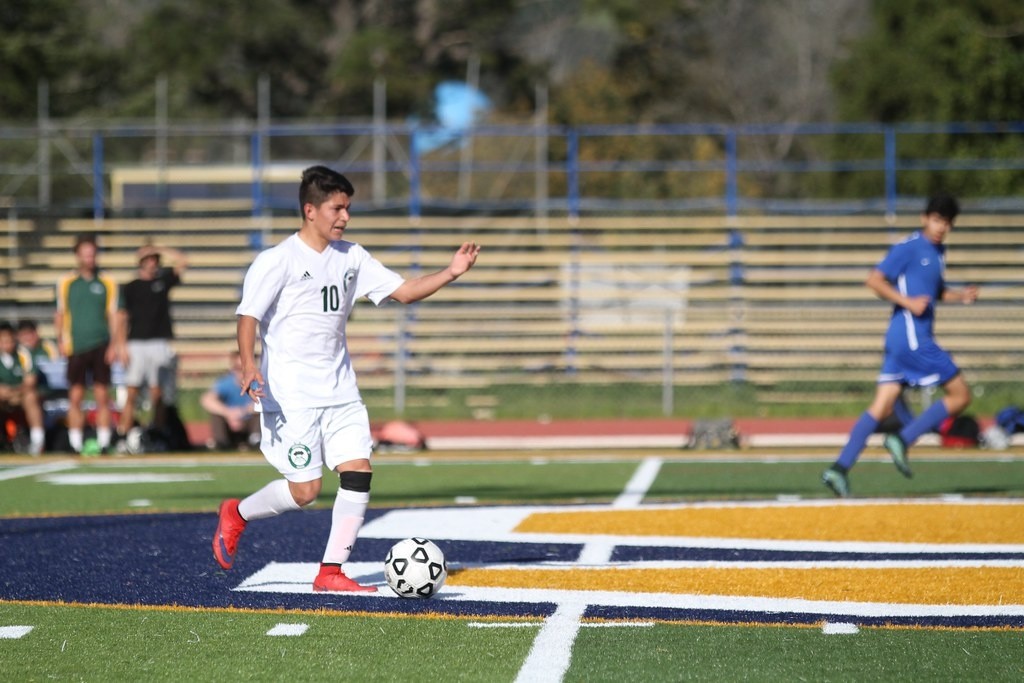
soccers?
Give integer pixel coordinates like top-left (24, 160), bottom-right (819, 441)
top-left (384, 537), bottom-right (448, 599)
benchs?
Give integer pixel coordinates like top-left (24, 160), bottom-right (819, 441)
top-left (0, 213), bottom-right (1024, 389)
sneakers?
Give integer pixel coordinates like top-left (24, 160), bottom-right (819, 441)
top-left (822, 470), bottom-right (850, 499)
top-left (885, 435), bottom-right (912, 479)
top-left (313, 567), bottom-right (378, 592)
top-left (210, 498), bottom-right (249, 569)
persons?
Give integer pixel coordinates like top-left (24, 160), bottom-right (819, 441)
top-left (0, 319), bottom-right (56, 459)
top-left (53, 231), bottom-right (265, 457)
top-left (822, 188), bottom-right (980, 500)
top-left (213, 165), bottom-right (482, 594)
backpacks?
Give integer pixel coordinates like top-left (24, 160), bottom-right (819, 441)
top-left (140, 405), bottom-right (190, 452)
